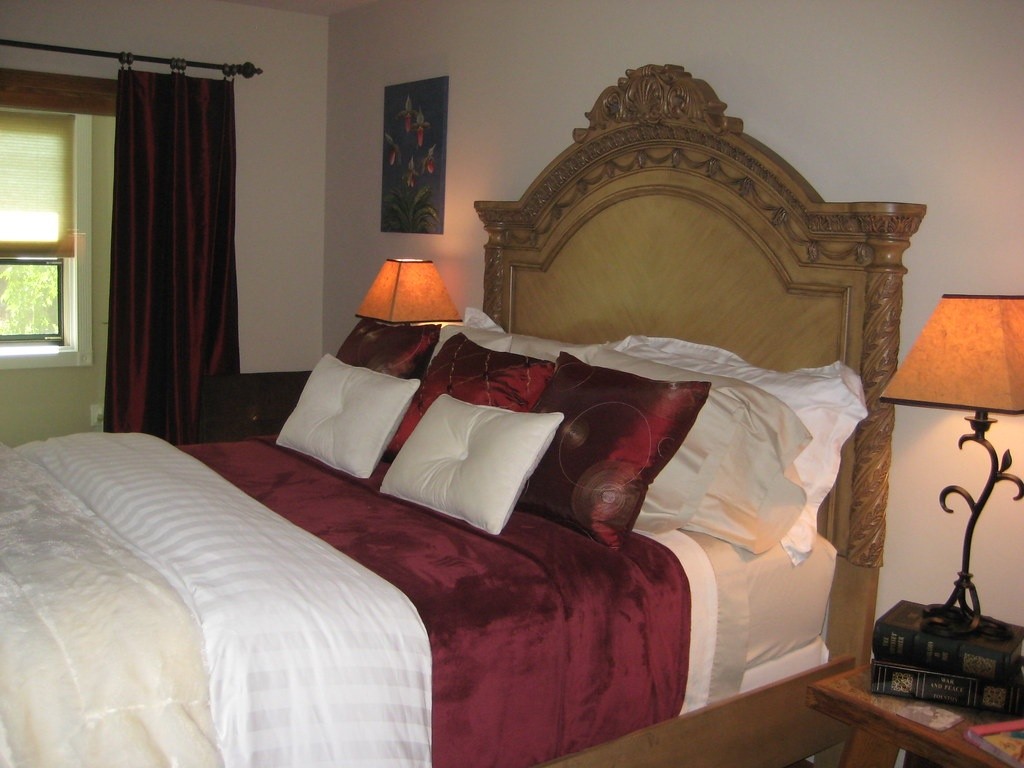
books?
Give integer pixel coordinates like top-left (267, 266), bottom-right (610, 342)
top-left (962, 719), bottom-right (1024, 768)
top-left (869, 600), bottom-right (1024, 715)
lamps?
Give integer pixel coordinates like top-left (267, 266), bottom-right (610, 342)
top-left (879, 294), bottom-right (1024, 640)
top-left (355, 257), bottom-right (463, 326)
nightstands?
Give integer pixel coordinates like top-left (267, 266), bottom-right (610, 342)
top-left (805, 663), bottom-right (1024, 767)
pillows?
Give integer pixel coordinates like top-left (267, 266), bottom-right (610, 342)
top-left (428, 308), bottom-right (608, 365)
top-left (276, 353), bottom-right (420, 479)
top-left (335, 319), bottom-right (442, 379)
top-left (515, 351), bottom-right (711, 549)
top-left (382, 332), bottom-right (555, 463)
top-left (590, 336), bottom-right (868, 566)
top-left (380, 393), bottom-right (565, 535)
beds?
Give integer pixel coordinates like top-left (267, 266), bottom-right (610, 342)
top-left (0, 63), bottom-right (926, 766)
